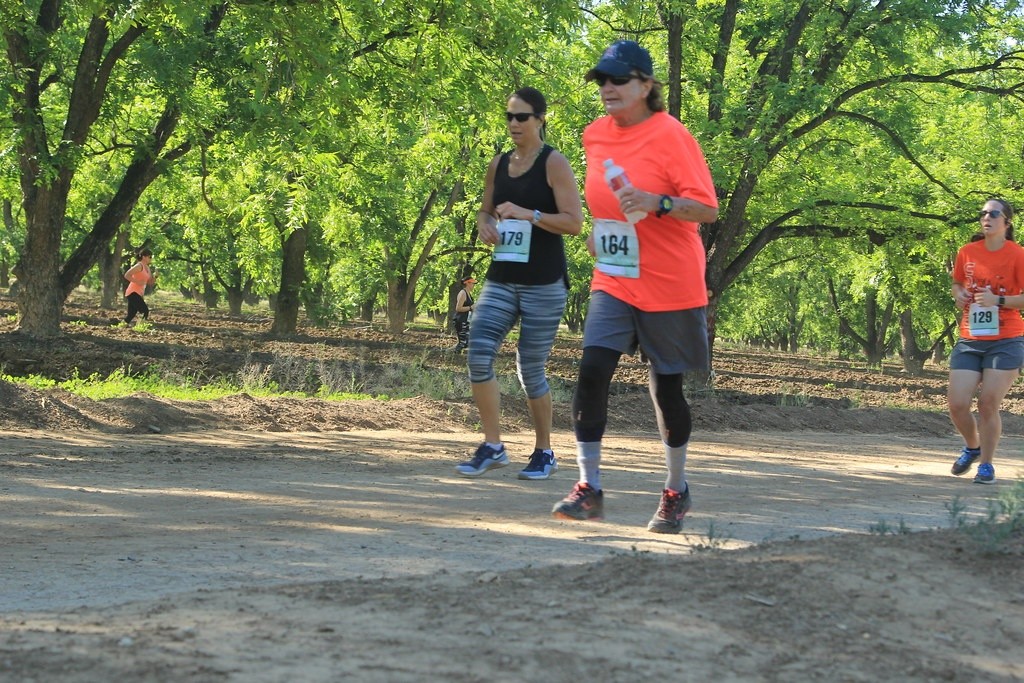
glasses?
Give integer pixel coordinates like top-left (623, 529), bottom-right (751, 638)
top-left (505, 112), bottom-right (543, 122)
top-left (596, 72), bottom-right (647, 86)
top-left (979, 210), bottom-right (1008, 220)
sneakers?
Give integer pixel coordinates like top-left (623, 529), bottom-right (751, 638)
top-left (518, 447), bottom-right (557, 480)
top-left (649, 481), bottom-right (691, 533)
top-left (552, 481), bottom-right (605, 521)
top-left (973, 463), bottom-right (996, 484)
top-left (455, 442), bottom-right (509, 475)
top-left (951, 446), bottom-right (981, 476)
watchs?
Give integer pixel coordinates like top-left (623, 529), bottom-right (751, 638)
top-left (532, 210), bottom-right (541, 224)
top-left (997, 296), bottom-right (1005, 307)
top-left (656, 193), bottom-right (673, 217)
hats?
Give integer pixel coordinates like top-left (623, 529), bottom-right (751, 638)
top-left (584, 40), bottom-right (654, 83)
top-left (463, 278), bottom-right (478, 284)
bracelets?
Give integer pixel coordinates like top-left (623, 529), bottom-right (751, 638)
top-left (470, 306), bottom-right (471, 310)
top-left (155, 276), bottom-right (157, 278)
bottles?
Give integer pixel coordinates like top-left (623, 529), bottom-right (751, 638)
top-left (601, 159), bottom-right (647, 224)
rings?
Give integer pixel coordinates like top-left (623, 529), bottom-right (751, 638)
top-left (627, 201), bottom-right (632, 208)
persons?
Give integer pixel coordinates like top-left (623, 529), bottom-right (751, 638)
top-left (550, 40), bottom-right (719, 534)
top-left (121, 249), bottom-right (159, 322)
top-left (457, 87), bottom-right (582, 480)
top-left (450, 276), bottom-right (478, 353)
top-left (948, 199), bottom-right (1024, 483)
